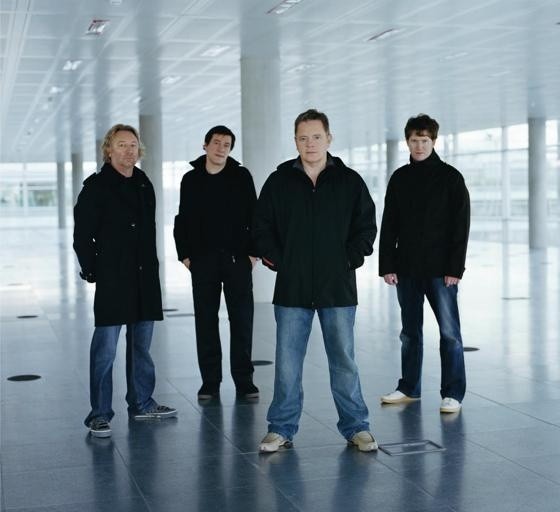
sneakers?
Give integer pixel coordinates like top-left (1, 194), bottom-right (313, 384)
top-left (134, 404), bottom-right (177, 419)
top-left (439, 395), bottom-right (463, 413)
top-left (380, 388), bottom-right (422, 404)
top-left (257, 428), bottom-right (290, 452)
top-left (351, 429), bottom-right (378, 453)
top-left (196, 382), bottom-right (220, 399)
top-left (236, 380), bottom-right (260, 398)
top-left (89, 417), bottom-right (112, 439)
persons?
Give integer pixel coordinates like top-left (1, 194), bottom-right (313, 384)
top-left (244, 108), bottom-right (381, 455)
top-left (375, 112), bottom-right (475, 415)
top-left (67, 121), bottom-right (182, 439)
top-left (166, 124), bottom-right (262, 402)
